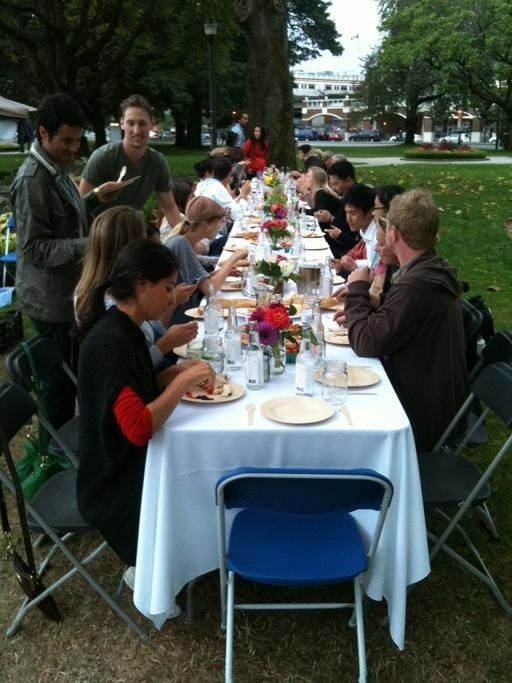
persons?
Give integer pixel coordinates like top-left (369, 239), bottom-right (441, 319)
top-left (79, 94), bottom-right (185, 218)
top-left (72, 113), bottom-right (268, 369)
top-left (294, 145), bottom-right (409, 328)
top-left (295, 128), bottom-right (471, 144)
top-left (333, 189), bottom-right (471, 451)
top-left (75, 239), bottom-right (215, 618)
top-left (7, 95), bottom-right (120, 442)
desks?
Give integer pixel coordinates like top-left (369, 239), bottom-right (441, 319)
top-left (234, 172), bottom-right (316, 214)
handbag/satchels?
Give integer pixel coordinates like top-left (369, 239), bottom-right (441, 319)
top-left (16, 434), bottom-right (73, 501)
top-left (13, 551), bottom-right (63, 623)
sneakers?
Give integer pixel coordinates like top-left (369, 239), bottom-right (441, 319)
top-left (122, 566), bottom-right (182, 619)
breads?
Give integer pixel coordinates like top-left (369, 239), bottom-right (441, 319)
top-left (209, 292), bottom-right (337, 308)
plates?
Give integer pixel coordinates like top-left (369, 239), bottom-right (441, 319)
top-left (181, 383), bottom-right (245, 403)
top-left (333, 276), bottom-right (347, 285)
top-left (261, 395), bottom-right (335, 424)
top-left (303, 242), bottom-right (329, 250)
top-left (184, 307), bottom-right (230, 320)
top-left (313, 367), bottom-right (381, 388)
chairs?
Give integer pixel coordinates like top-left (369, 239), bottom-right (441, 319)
top-left (0, 215), bottom-right (512, 683)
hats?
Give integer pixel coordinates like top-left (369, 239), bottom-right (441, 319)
top-left (185, 196), bottom-right (226, 220)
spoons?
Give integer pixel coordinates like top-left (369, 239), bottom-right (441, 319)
top-left (245, 404), bottom-right (255, 425)
top-left (117, 166), bottom-right (126, 183)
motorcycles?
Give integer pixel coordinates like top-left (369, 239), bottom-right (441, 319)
top-left (392, 136), bottom-right (405, 144)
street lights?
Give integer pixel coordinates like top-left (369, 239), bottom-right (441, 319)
top-left (203, 14), bottom-right (218, 148)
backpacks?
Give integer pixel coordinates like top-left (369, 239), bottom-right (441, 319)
top-left (467, 295), bottom-right (494, 357)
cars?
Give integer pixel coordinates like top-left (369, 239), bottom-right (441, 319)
top-left (390, 132), bottom-right (423, 143)
top-left (319, 130), bottom-right (342, 141)
top-left (349, 129), bottom-right (380, 142)
top-left (439, 133), bottom-right (471, 145)
top-left (161, 130), bottom-right (171, 140)
top-left (294, 129), bottom-right (318, 142)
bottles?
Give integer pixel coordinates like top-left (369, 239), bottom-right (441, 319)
top-left (187, 245), bottom-right (263, 390)
top-left (296, 300), bottom-right (348, 403)
top-left (320, 256), bottom-right (332, 298)
top-left (280, 166), bottom-right (291, 182)
top-left (289, 197), bottom-right (300, 230)
top-left (266, 343), bottom-right (287, 373)
